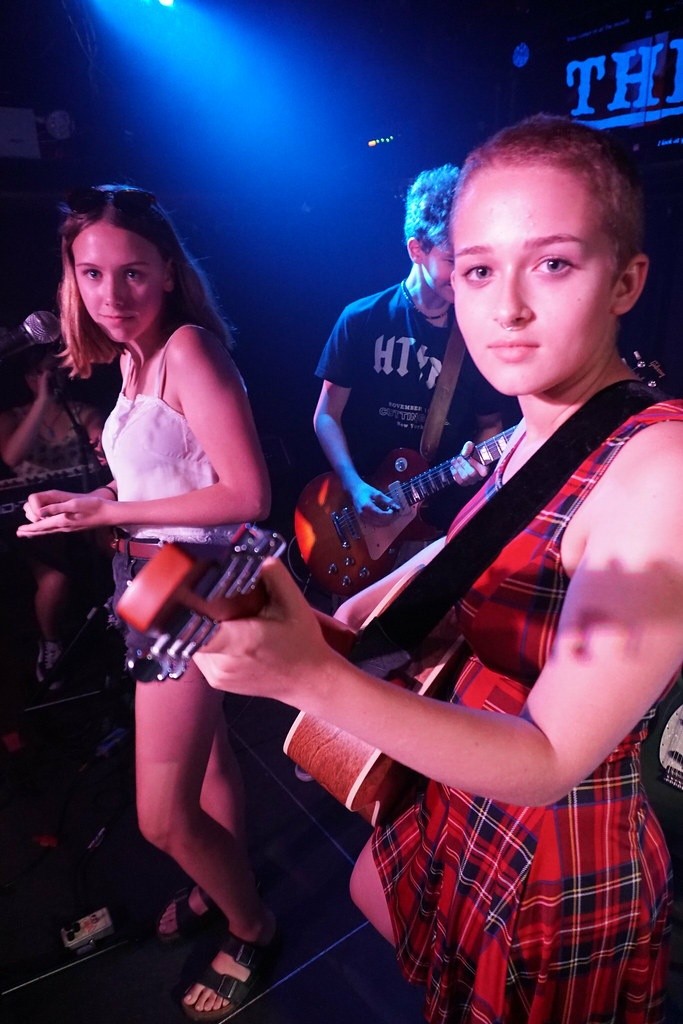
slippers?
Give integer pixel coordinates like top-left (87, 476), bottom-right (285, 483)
top-left (154, 874), bottom-right (264, 944)
top-left (182, 918), bottom-right (284, 1022)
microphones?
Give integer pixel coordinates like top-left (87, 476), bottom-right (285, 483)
top-left (0, 312), bottom-right (62, 360)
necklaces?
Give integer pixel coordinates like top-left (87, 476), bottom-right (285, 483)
top-left (41, 407), bottom-right (62, 441)
top-left (401, 279), bottom-right (449, 320)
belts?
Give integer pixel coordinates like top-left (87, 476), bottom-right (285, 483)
top-left (110, 537), bottom-right (159, 560)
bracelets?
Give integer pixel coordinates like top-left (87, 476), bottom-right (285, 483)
top-left (97, 486), bottom-right (118, 500)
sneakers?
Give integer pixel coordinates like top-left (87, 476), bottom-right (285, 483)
top-left (36, 635), bottom-right (65, 690)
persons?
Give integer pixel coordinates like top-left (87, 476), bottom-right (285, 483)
top-left (0, 325), bottom-right (115, 689)
top-left (169, 115), bottom-right (683, 1024)
top-left (13, 186), bottom-right (286, 1019)
top-left (313, 166), bottom-right (503, 579)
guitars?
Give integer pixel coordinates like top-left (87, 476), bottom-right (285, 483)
top-left (113, 512), bottom-right (471, 830)
top-left (295, 345), bottom-right (663, 601)
